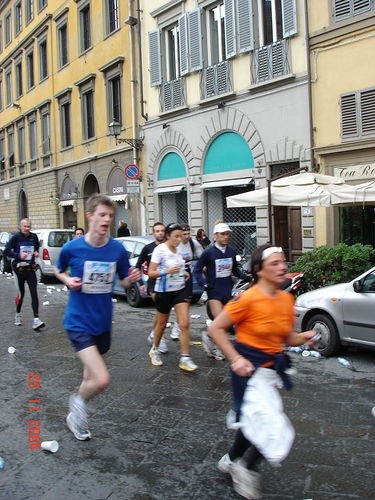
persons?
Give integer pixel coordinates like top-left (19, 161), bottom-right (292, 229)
top-left (75, 228), bottom-right (84, 237)
top-left (207, 244), bottom-right (318, 500)
top-left (56, 191), bottom-right (141, 440)
top-left (148, 223), bottom-right (199, 370)
top-left (4, 219), bottom-right (46, 329)
top-left (193, 221), bottom-right (253, 360)
top-left (117, 220), bottom-right (130, 237)
top-left (171, 223), bottom-right (203, 339)
top-left (196, 229), bottom-right (212, 249)
top-left (135, 222), bottom-right (169, 353)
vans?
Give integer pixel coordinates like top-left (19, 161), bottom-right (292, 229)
top-left (29, 229), bottom-right (79, 284)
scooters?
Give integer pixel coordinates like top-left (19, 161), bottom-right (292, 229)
top-left (226, 254), bottom-right (304, 300)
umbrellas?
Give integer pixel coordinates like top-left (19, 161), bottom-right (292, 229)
top-left (330, 180), bottom-right (375, 204)
top-left (226, 166), bottom-right (354, 245)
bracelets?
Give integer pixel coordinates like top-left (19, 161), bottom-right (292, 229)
top-left (229, 355), bottom-right (242, 364)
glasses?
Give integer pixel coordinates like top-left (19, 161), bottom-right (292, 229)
top-left (169, 223), bottom-right (180, 230)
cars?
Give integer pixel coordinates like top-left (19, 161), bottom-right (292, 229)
top-left (109, 235), bottom-right (208, 308)
top-left (0, 232), bottom-right (21, 272)
top-left (294, 267), bottom-right (375, 357)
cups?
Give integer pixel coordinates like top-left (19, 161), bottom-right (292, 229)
top-left (43, 301), bottom-right (49, 306)
top-left (302, 350), bottom-right (320, 357)
top-left (191, 314), bottom-right (198, 319)
top-left (339, 358), bottom-right (349, 365)
top-left (8, 346), bottom-right (16, 354)
top-left (41, 440), bottom-right (59, 453)
top-left (165, 323), bottom-right (171, 328)
top-left (112, 298), bottom-right (117, 303)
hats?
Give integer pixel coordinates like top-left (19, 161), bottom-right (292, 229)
top-left (214, 223), bottom-right (233, 235)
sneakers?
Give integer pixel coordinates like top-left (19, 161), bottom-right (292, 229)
top-left (170, 328), bottom-right (181, 339)
top-left (149, 347), bottom-right (163, 365)
top-left (148, 331), bottom-right (168, 353)
top-left (33, 319), bottom-right (45, 330)
top-left (179, 358), bottom-right (198, 371)
top-left (218, 453), bottom-right (239, 473)
top-left (202, 331), bottom-right (212, 352)
top-left (207, 349), bottom-right (225, 360)
top-left (67, 411), bottom-right (92, 440)
top-left (230, 462), bottom-right (261, 500)
top-left (15, 312), bottom-right (22, 325)
